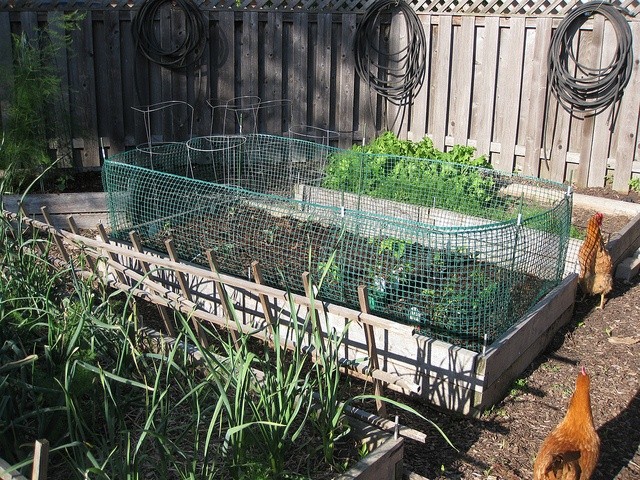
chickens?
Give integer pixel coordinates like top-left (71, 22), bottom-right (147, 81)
top-left (575, 213), bottom-right (615, 310)
top-left (534, 366), bottom-right (601, 480)
